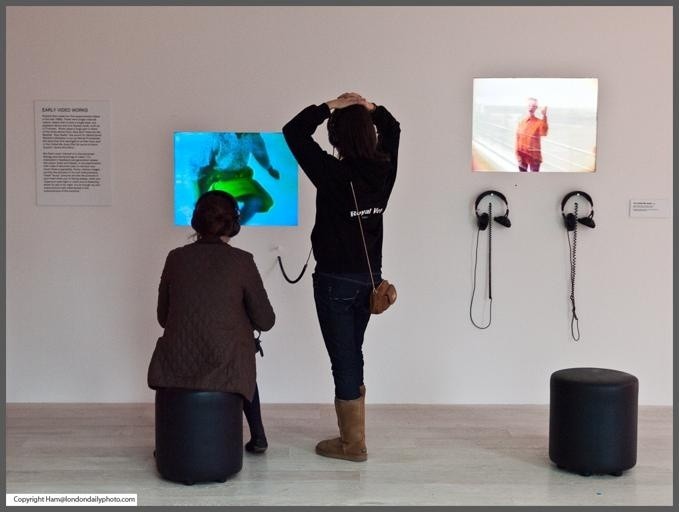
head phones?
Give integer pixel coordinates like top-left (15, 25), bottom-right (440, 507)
top-left (191, 191), bottom-right (240, 238)
top-left (328, 108), bottom-right (342, 148)
top-left (561, 191), bottom-right (595, 230)
top-left (475, 190), bottom-right (511, 230)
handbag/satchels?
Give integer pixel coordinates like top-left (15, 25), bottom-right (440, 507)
top-left (370, 280), bottom-right (397, 314)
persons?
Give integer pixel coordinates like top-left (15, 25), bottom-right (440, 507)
top-left (284, 93), bottom-right (403, 460)
top-left (515, 100), bottom-right (549, 172)
top-left (147, 191), bottom-right (276, 453)
top-left (192, 132), bottom-right (279, 225)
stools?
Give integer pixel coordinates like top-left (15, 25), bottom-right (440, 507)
top-left (154, 388), bottom-right (243, 486)
top-left (550, 367), bottom-right (638, 477)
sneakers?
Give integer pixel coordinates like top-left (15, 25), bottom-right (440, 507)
top-left (246, 436), bottom-right (269, 454)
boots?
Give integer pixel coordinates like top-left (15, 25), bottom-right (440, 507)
top-left (316, 384), bottom-right (367, 462)
